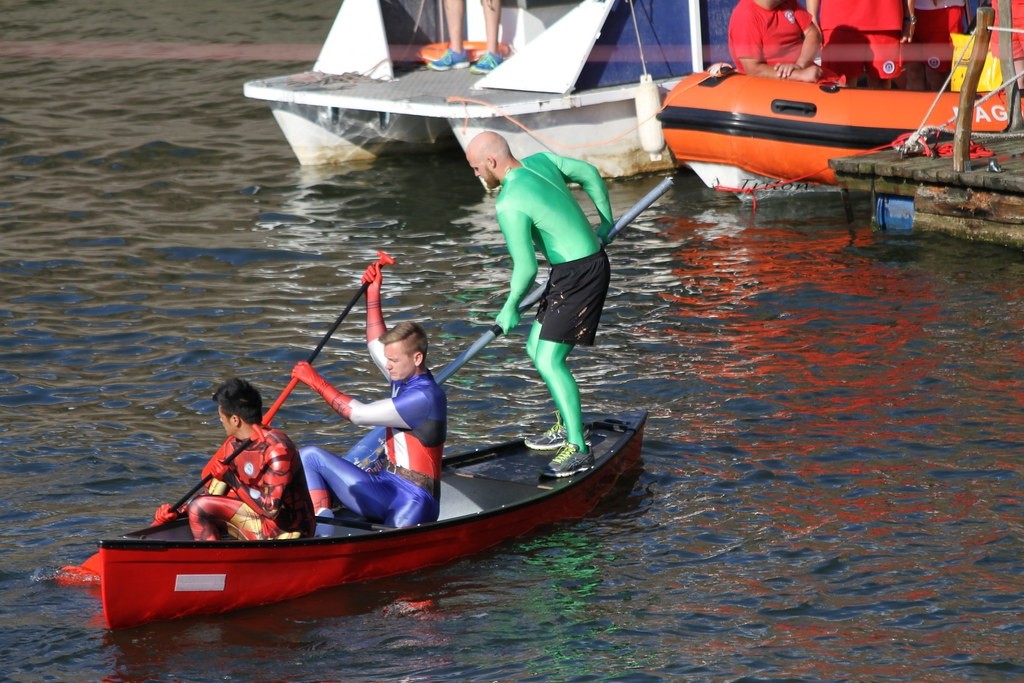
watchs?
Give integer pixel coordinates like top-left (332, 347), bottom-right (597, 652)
top-left (905, 17), bottom-right (917, 23)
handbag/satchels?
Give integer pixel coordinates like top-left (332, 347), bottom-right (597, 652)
top-left (949, 32), bottom-right (1003, 93)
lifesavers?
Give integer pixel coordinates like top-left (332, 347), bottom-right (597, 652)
top-left (417, 41), bottom-right (511, 66)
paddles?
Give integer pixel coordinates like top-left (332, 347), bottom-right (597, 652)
top-left (81, 423), bottom-right (265, 573)
top-left (201, 251), bottom-right (395, 488)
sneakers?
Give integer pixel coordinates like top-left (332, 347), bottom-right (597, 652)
top-left (470, 53), bottom-right (503, 74)
top-left (427, 47), bottom-right (470, 71)
top-left (539, 441), bottom-right (595, 477)
top-left (525, 410), bottom-right (589, 451)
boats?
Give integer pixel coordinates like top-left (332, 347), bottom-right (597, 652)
top-left (656, 71), bottom-right (1024, 202)
top-left (98, 407), bottom-right (651, 629)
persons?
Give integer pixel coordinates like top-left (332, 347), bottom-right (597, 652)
top-left (465, 131), bottom-right (615, 477)
top-left (154, 378), bottom-right (315, 542)
top-left (728, 0), bottom-right (839, 84)
top-left (292, 260), bottom-right (448, 527)
top-left (426, 0), bottom-right (503, 75)
top-left (805, 0), bottom-right (1024, 95)
